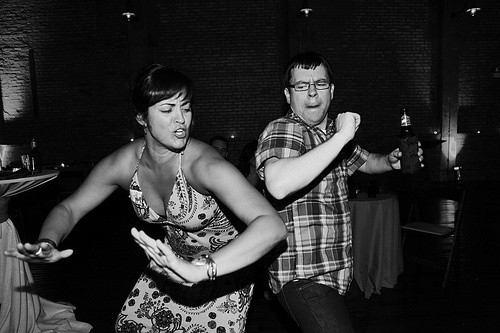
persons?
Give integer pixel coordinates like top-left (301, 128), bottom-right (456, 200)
top-left (3, 57), bottom-right (426, 332)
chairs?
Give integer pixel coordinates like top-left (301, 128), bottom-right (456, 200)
top-left (400, 184), bottom-right (469, 289)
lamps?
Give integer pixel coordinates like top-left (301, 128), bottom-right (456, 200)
top-left (466, 0), bottom-right (482, 17)
top-left (301, 0), bottom-right (313, 18)
top-left (120, 1), bottom-right (137, 21)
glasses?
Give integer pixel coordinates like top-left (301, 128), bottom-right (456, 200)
top-left (287, 81), bottom-right (331, 91)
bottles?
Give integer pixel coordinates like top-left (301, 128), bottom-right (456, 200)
top-left (399, 108), bottom-right (424, 174)
top-left (29, 136), bottom-right (42, 176)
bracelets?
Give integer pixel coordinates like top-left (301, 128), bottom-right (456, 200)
top-left (204, 255), bottom-right (217, 281)
top-left (36, 238), bottom-right (57, 249)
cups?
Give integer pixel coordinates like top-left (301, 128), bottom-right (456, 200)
top-left (20, 155), bottom-right (31, 175)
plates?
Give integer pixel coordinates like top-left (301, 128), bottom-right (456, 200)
top-left (0, 167), bottom-right (21, 174)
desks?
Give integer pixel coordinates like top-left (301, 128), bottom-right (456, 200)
top-left (0, 169), bottom-right (60, 332)
top-left (349, 193), bottom-right (404, 299)
